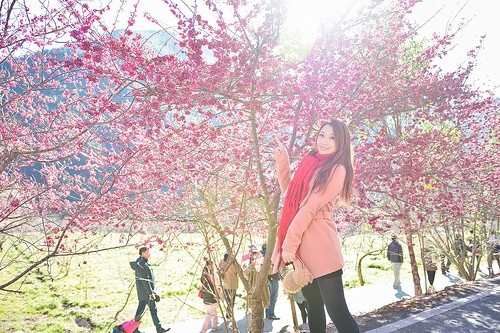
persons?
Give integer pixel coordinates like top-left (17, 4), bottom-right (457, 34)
top-left (420, 238), bottom-right (439, 289)
top-left (441, 230), bottom-right (500, 278)
top-left (129, 246), bottom-right (171, 333)
top-left (198, 241), bottom-right (309, 333)
top-left (387, 234), bottom-right (404, 289)
top-left (273, 119), bottom-right (361, 333)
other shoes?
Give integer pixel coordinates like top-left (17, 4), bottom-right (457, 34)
top-left (268, 314), bottom-right (280, 320)
top-left (156, 327), bottom-right (171, 333)
top-left (134, 328), bottom-right (141, 333)
